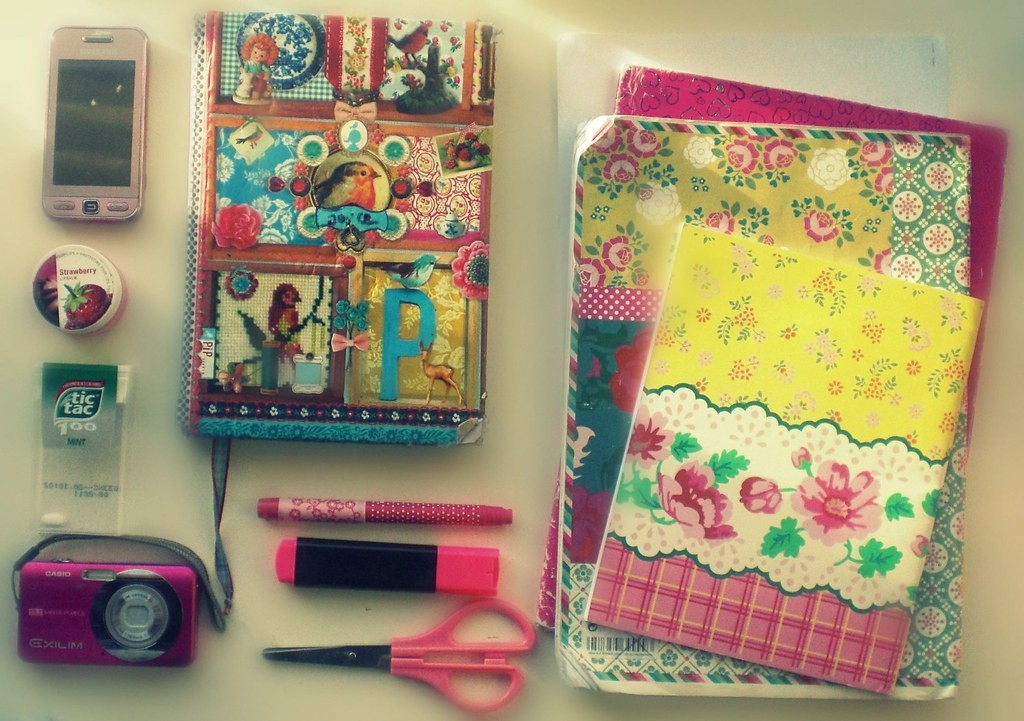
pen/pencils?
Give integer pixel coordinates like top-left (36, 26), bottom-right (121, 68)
top-left (257, 495), bottom-right (514, 527)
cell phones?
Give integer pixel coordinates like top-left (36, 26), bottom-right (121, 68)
top-left (43, 26), bottom-right (150, 221)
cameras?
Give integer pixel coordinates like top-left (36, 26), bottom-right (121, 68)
top-left (18, 558), bottom-right (201, 668)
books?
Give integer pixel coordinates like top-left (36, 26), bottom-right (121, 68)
top-left (535, 63), bottom-right (1009, 703)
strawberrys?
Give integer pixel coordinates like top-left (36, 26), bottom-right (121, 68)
top-left (61, 281), bottom-right (109, 328)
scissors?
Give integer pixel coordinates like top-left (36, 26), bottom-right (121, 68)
top-left (260, 595), bottom-right (538, 715)
